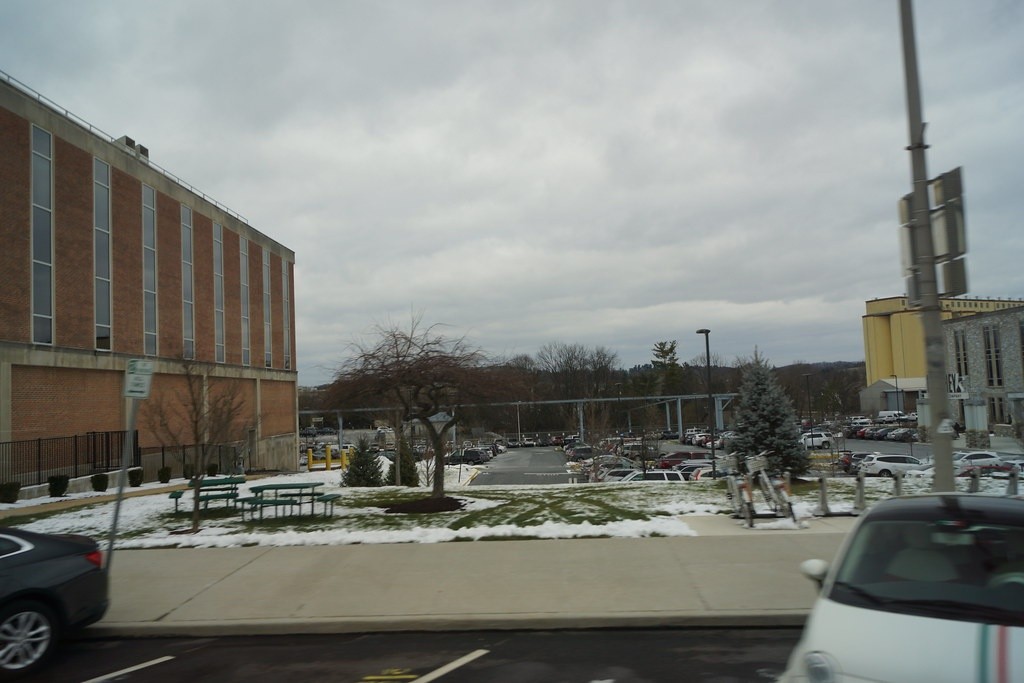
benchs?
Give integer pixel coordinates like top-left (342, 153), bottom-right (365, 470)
top-left (280, 492), bottom-right (324, 517)
top-left (194, 493), bottom-right (239, 511)
top-left (249, 500), bottom-right (298, 520)
top-left (198, 487), bottom-right (238, 495)
top-left (316, 493), bottom-right (342, 519)
top-left (233, 496), bottom-right (258, 519)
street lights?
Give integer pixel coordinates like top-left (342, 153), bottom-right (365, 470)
top-left (890, 374), bottom-right (901, 427)
top-left (801, 374), bottom-right (817, 449)
top-left (696, 328), bottom-right (717, 478)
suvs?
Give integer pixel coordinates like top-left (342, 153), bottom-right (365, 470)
top-left (860, 454), bottom-right (929, 478)
top-left (876, 415), bottom-right (895, 424)
top-left (797, 432), bottom-right (836, 450)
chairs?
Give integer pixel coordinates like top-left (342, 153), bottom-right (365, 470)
top-left (885, 525), bottom-right (962, 584)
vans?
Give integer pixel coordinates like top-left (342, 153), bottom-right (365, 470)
top-left (879, 411), bottom-right (909, 422)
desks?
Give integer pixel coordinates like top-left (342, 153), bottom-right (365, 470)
top-left (249, 482), bottom-right (325, 517)
top-left (188, 477), bottom-right (245, 505)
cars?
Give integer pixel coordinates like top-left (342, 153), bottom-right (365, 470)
top-left (802, 415), bottom-right (927, 442)
top-left (907, 413), bottom-right (918, 421)
top-left (838, 451), bottom-right (1024, 479)
top-left (0, 527), bottom-right (110, 683)
top-left (299, 428), bottom-right (741, 482)
